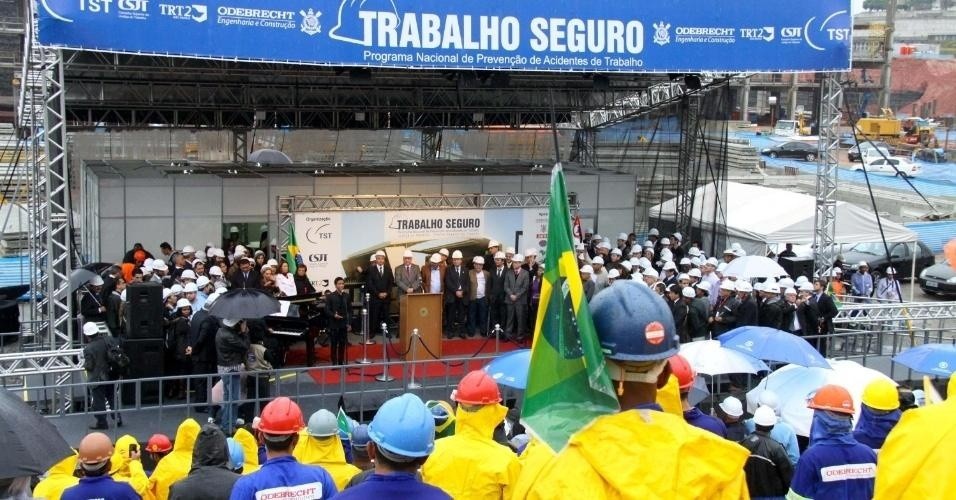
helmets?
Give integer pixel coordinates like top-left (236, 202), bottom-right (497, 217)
top-left (133, 246), bottom-right (225, 308)
top-left (428, 248), bottom-right (463, 263)
top-left (258, 396), bottom-right (306, 435)
top-left (487, 240), bottom-right (538, 262)
top-left (230, 226), bottom-right (238, 232)
top-left (307, 409), bottom-right (339, 438)
top-left (267, 259), bottom-right (279, 266)
top-left (719, 275), bottom-right (815, 294)
top-left (806, 384), bottom-right (856, 413)
top-left (351, 392), bottom-right (436, 458)
top-left (370, 250), bottom-right (386, 261)
top-left (402, 251), bottom-right (413, 257)
top-left (225, 437), bottom-right (244, 470)
top-left (859, 379), bottom-right (900, 411)
top-left (454, 369), bottom-right (502, 404)
top-left (576, 227), bottom-right (718, 298)
top-left (588, 280), bottom-right (695, 392)
top-left (472, 256), bottom-right (485, 265)
top-left (89, 274), bottom-right (104, 286)
top-left (720, 391), bottom-right (782, 426)
top-left (145, 433), bottom-right (173, 452)
top-left (79, 433), bottom-right (116, 465)
top-left (82, 321), bottom-right (99, 336)
top-left (716, 242), bottom-right (746, 272)
top-left (833, 262), bottom-right (896, 274)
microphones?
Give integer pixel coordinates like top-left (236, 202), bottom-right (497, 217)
top-left (341, 290), bottom-right (345, 295)
top-left (418, 276), bottom-right (423, 285)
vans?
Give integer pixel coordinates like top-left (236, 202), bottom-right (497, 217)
top-left (774, 119), bottom-right (800, 137)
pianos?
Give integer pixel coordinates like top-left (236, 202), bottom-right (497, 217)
top-left (263, 316), bottom-right (312, 369)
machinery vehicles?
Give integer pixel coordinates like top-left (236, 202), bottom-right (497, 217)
top-left (798, 111), bottom-right (811, 136)
top-left (854, 108), bottom-right (941, 155)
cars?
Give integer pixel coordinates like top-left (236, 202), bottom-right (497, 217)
top-left (919, 257), bottom-right (956, 298)
top-left (847, 141), bottom-right (895, 162)
top-left (861, 147), bottom-right (891, 166)
top-left (849, 157), bottom-right (924, 180)
top-left (825, 238), bottom-right (935, 288)
top-left (760, 141), bottom-right (823, 162)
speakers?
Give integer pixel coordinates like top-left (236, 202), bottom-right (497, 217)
top-left (122, 339), bottom-right (164, 404)
top-left (779, 256), bottom-right (814, 282)
top-left (127, 282), bottom-right (163, 338)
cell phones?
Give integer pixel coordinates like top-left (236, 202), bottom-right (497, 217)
top-left (129, 443), bottom-right (136, 457)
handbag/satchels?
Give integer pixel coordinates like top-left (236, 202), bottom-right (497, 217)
top-left (106, 345), bottom-right (132, 378)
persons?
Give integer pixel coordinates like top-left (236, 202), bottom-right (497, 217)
top-left (0, 223), bottom-right (956, 500)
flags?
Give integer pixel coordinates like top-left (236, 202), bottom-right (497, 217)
top-left (515, 162), bottom-right (624, 460)
